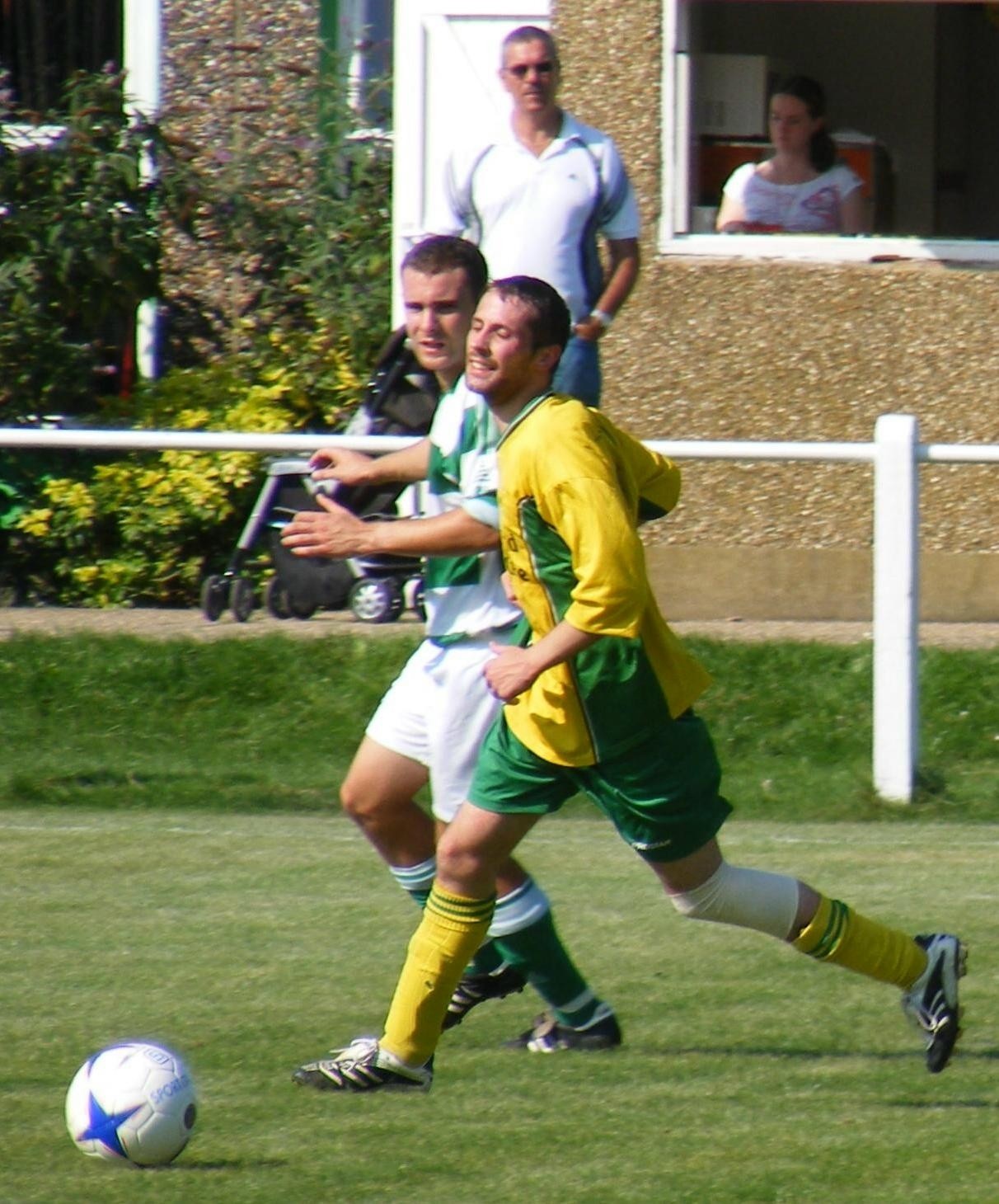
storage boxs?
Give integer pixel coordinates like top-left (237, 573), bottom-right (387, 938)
top-left (694, 54), bottom-right (770, 136)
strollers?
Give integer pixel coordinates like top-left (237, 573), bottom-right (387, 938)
top-left (200, 320), bottom-right (446, 626)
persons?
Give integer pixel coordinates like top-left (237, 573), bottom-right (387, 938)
top-left (421, 25), bottom-right (645, 410)
top-left (714, 75), bottom-right (868, 239)
top-left (291, 276), bottom-right (969, 1092)
top-left (281, 236), bottom-right (622, 1057)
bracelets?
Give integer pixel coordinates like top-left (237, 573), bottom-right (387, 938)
top-left (588, 310), bottom-right (614, 329)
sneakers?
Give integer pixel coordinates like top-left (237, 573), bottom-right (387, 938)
top-left (291, 1038), bottom-right (436, 1094)
top-left (504, 998), bottom-right (621, 1055)
top-left (901, 934), bottom-right (967, 1074)
top-left (440, 962), bottom-right (529, 1029)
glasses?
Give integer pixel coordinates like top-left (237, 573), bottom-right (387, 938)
top-left (504, 62), bottom-right (553, 75)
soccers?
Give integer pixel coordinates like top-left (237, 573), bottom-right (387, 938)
top-left (63, 1039), bottom-right (198, 1168)
